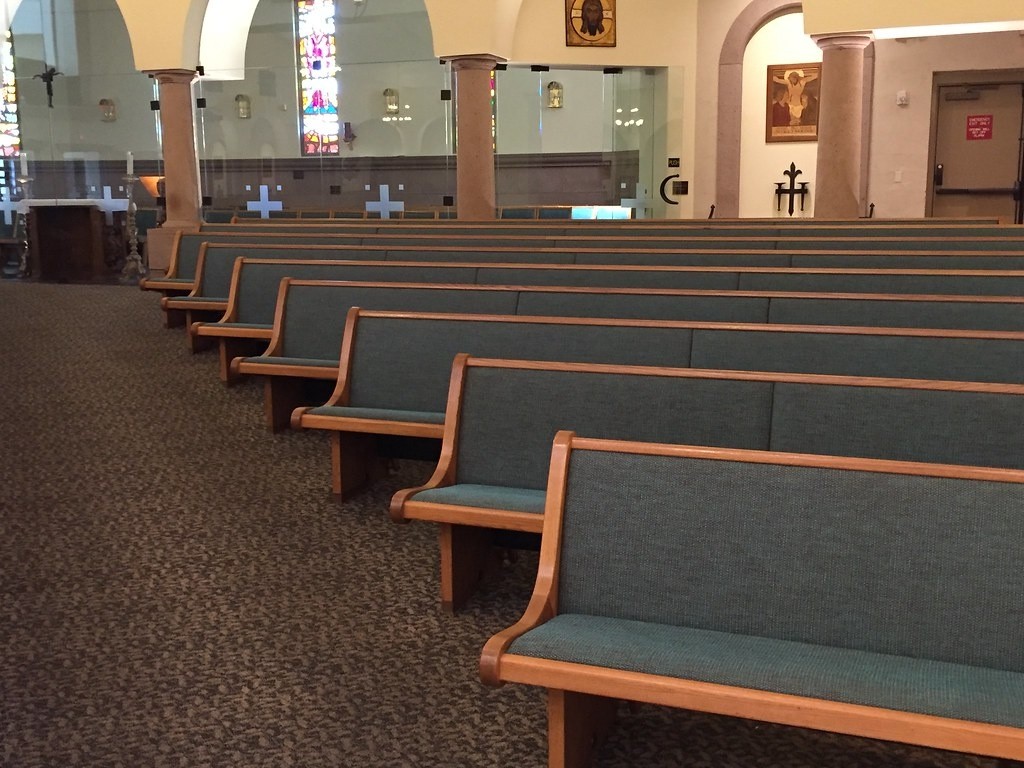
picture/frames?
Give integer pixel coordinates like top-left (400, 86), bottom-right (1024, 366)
top-left (765, 62), bottom-right (823, 142)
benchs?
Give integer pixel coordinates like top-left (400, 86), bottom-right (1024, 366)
top-left (140, 207), bottom-right (1024, 768)
